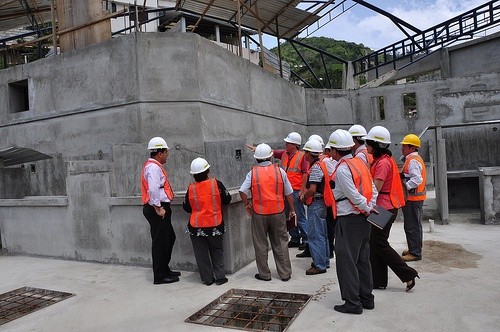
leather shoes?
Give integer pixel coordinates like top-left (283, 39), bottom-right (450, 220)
top-left (154, 270), bottom-right (181, 284)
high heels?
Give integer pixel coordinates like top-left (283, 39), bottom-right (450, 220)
top-left (406, 274), bottom-right (420, 291)
top-left (373, 285), bottom-right (387, 289)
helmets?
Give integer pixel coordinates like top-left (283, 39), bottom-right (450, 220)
top-left (147, 137), bottom-right (169, 149)
top-left (360, 125), bottom-right (391, 144)
top-left (324, 129), bottom-right (355, 149)
top-left (189, 158), bottom-right (211, 175)
top-left (283, 132), bottom-right (301, 145)
top-left (309, 135), bottom-right (324, 146)
top-left (347, 124), bottom-right (367, 137)
top-left (303, 140), bottom-right (323, 152)
top-left (400, 134), bottom-right (421, 148)
top-left (254, 144), bottom-right (273, 159)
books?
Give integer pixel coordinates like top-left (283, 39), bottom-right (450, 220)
top-left (367, 206), bottom-right (394, 230)
top-left (286, 215), bottom-right (296, 231)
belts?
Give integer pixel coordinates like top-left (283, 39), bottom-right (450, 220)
top-left (312, 197), bottom-right (324, 200)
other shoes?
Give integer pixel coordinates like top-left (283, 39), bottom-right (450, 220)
top-left (402, 250), bottom-right (410, 256)
top-left (400, 254), bottom-right (422, 261)
top-left (334, 300), bottom-right (374, 314)
top-left (306, 262), bottom-right (326, 275)
top-left (298, 244), bottom-right (306, 250)
top-left (255, 274), bottom-right (271, 281)
top-left (206, 278), bottom-right (215, 286)
top-left (282, 276), bottom-right (291, 281)
top-left (296, 249), bottom-right (312, 257)
top-left (288, 241), bottom-right (300, 247)
top-left (216, 278), bottom-right (228, 285)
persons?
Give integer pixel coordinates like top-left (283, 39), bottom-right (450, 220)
top-left (329, 129), bottom-right (379, 314)
top-left (141, 137), bottom-right (182, 285)
top-left (244, 132), bottom-right (308, 249)
top-left (238, 142), bottom-right (295, 281)
top-left (297, 124), bottom-right (426, 276)
top-left (183, 157), bottom-right (232, 285)
top-left (360, 125), bottom-right (421, 292)
